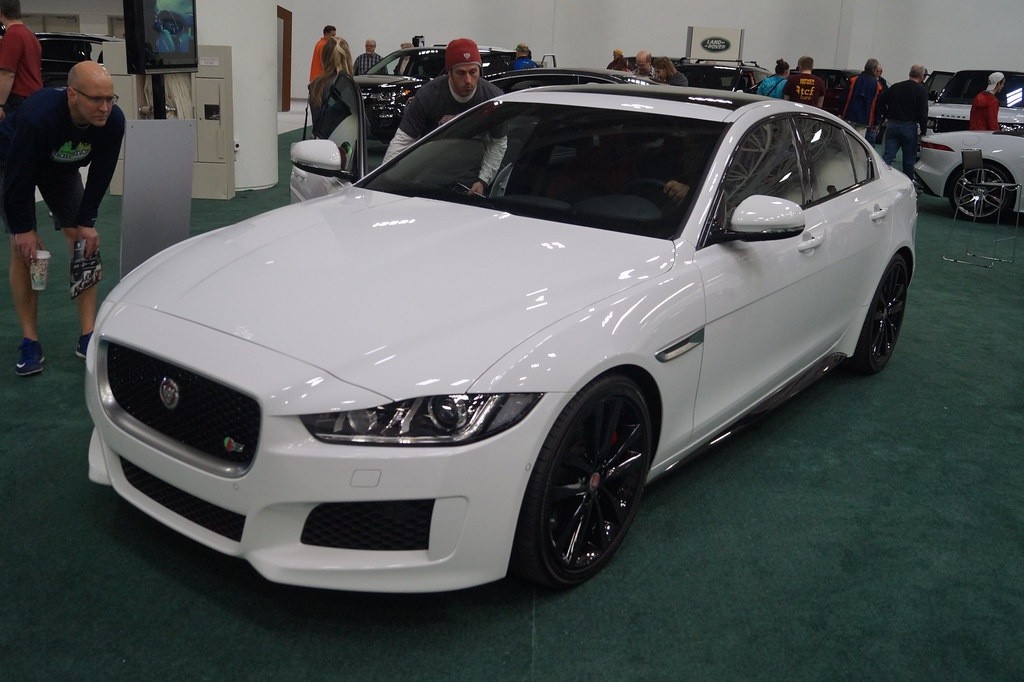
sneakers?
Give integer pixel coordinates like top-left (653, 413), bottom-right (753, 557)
top-left (15, 342), bottom-right (45, 375)
top-left (74, 334), bottom-right (92, 359)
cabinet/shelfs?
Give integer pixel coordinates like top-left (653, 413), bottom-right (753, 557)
top-left (96, 38), bottom-right (234, 201)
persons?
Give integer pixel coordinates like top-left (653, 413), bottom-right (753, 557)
top-left (308, 36), bottom-right (354, 138)
top-left (607, 49), bottom-right (635, 73)
top-left (654, 56), bottom-right (688, 87)
top-left (0, 0), bottom-right (44, 118)
top-left (383, 38), bottom-right (507, 197)
top-left (632, 50), bottom-right (656, 77)
top-left (757, 59), bottom-right (790, 99)
top-left (310, 25), bottom-right (336, 82)
top-left (840, 58), bottom-right (891, 149)
top-left (509, 43), bottom-right (538, 70)
top-left (400, 43), bottom-right (414, 75)
top-left (353, 40), bottom-right (389, 74)
top-left (0, 61), bottom-right (126, 376)
top-left (968, 72), bottom-right (1005, 131)
top-left (882, 64), bottom-right (928, 179)
top-left (664, 180), bottom-right (689, 206)
top-left (782, 57), bottom-right (826, 107)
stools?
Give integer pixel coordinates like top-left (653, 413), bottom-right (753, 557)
top-left (939, 149), bottom-right (1023, 269)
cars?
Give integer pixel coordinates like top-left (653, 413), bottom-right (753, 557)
top-left (479, 66), bottom-right (668, 128)
top-left (910, 123), bottom-right (1024, 223)
top-left (0, 29), bottom-right (125, 89)
top-left (353, 42), bottom-right (559, 143)
top-left (85, 86), bottom-right (921, 594)
top-left (920, 69), bottom-right (1024, 137)
top-left (606, 56), bottom-right (890, 144)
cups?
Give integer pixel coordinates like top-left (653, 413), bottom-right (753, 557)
top-left (29, 250), bottom-right (50, 290)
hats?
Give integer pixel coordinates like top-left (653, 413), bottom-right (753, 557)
top-left (924, 69), bottom-right (930, 76)
top-left (613, 49), bottom-right (623, 57)
top-left (987, 72), bottom-right (1004, 90)
top-left (446, 38), bottom-right (482, 69)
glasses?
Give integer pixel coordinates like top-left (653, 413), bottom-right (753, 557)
top-left (635, 62), bottom-right (649, 68)
top-left (72, 88), bottom-right (119, 107)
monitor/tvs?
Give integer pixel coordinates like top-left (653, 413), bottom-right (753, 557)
top-left (137, 0), bottom-right (198, 74)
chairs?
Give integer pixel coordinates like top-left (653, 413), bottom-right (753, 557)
top-left (731, 77), bottom-right (747, 89)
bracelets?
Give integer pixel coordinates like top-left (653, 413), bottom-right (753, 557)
top-left (0, 105), bottom-right (4, 107)
